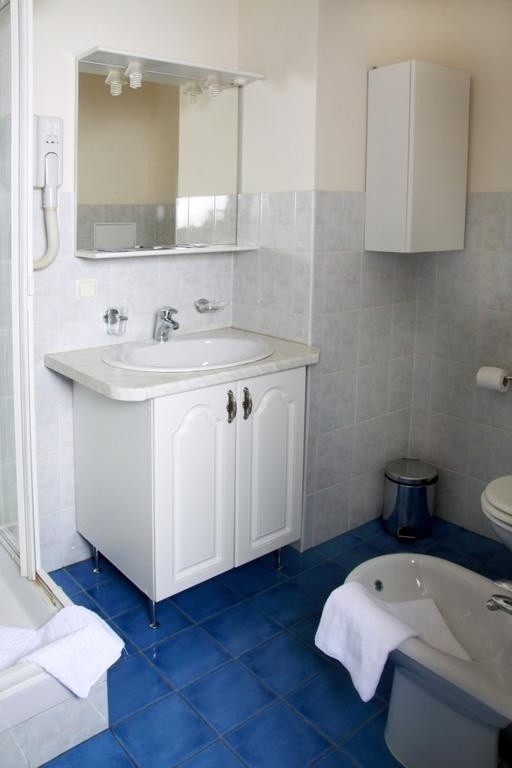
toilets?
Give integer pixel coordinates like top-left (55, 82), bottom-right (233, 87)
top-left (480, 475), bottom-right (512, 551)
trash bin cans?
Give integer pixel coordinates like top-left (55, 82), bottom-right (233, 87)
top-left (382, 457), bottom-right (438, 543)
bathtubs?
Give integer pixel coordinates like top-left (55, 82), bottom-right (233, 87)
top-left (1, 543), bottom-right (109, 768)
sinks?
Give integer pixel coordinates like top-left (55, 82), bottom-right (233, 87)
top-left (343, 552), bottom-right (511, 768)
top-left (103, 327), bottom-right (274, 373)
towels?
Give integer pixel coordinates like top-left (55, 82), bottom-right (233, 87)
top-left (1, 606), bottom-right (127, 700)
top-left (314, 583), bottom-right (473, 703)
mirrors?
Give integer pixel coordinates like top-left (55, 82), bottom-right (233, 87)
top-left (77, 56), bottom-right (243, 260)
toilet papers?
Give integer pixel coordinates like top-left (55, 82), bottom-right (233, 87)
top-left (475, 366), bottom-right (509, 393)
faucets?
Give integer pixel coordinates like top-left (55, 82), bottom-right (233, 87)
top-left (486, 595), bottom-right (512, 615)
top-left (154, 307), bottom-right (178, 342)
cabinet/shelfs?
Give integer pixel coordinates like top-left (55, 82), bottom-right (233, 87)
top-left (74, 367), bottom-right (307, 602)
top-left (369, 59), bottom-right (473, 252)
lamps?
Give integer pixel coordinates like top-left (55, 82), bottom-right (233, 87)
top-left (124, 61), bottom-right (149, 88)
top-left (105, 71), bottom-right (128, 96)
top-left (183, 80), bottom-right (205, 96)
top-left (203, 72), bottom-right (227, 97)
